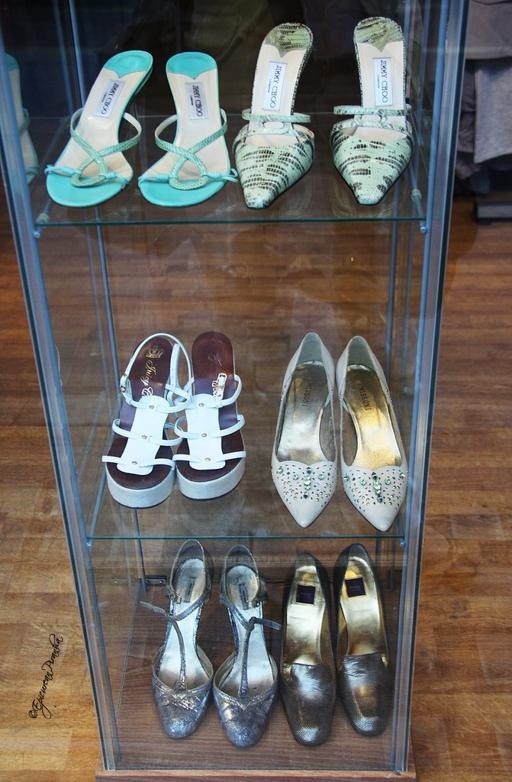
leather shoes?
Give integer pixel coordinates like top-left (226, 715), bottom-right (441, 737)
top-left (272, 332), bottom-right (408, 534)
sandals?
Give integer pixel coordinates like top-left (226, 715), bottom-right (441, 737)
top-left (102, 333), bottom-right (246, 509)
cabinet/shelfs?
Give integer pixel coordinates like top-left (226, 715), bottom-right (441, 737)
top-left (21, 113), bottom-right (421, 778)
